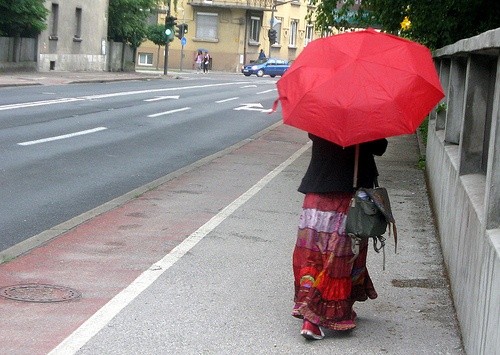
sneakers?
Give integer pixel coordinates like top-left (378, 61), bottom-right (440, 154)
top-left (301, 318), bottom-right (324, 339)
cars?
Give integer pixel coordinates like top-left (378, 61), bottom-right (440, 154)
top-left (242, 57), bottom-right (289, 78)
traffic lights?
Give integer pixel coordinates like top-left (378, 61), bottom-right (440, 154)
top-left (175, 24), bottom-right (182, 39)
top-left (164, 16), bottom-right (177, 37)
top-left (268, 29), bottom-right (277, 44)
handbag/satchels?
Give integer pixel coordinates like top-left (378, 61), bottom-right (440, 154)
top-left (345, 186), bottom-right (398, 270)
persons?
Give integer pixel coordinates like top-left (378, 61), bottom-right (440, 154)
top-left (194, 50), bottom-right (210, 74)
top-left (292, 132), bottom-right (388, 341)
top-left (259, 49), bottom-right (265, 58)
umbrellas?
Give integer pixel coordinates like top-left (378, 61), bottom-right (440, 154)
top-left (197, 48), bottom-right (208, 52)
top-left (268, 31), bottom-right (445, 147)
